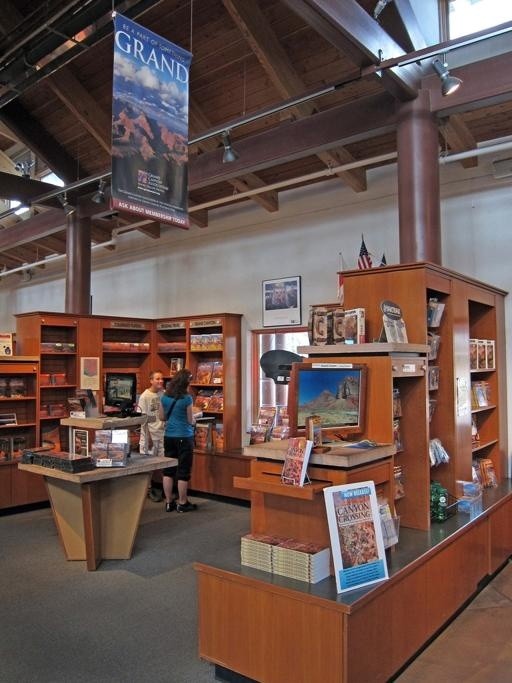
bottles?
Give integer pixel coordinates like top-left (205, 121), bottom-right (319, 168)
top-left (430, 478), bottom-right (450, 523)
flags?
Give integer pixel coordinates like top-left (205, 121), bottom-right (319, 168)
top-left (358, 241), bottom-right (374, 270)
top-left (337, 253), bottom-right (351, 300)
top-left (379, 252), bottom-right (386, 266)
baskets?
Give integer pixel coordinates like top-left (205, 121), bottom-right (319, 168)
top-left (240, 537), bottom-right (272, 573)
top-left (272, 544), bottom-right (330, 584)
top-left (431, 492), bottom-right (460, 521)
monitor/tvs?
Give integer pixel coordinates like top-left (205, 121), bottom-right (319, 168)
top-left (103, 371), bottom-right (136, 418)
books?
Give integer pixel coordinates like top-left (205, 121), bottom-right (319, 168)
top-left (1, 340), bottom-right (136, 474)
top-left (0, 331), bottom-right (14, 356)
top-left (170, 332), bottom-right (224, 451)
top-left (235, 299), bottom-right (496, 595)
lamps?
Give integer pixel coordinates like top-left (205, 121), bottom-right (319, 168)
top-left (55, 194), bottom-right (77, 217)
top-left (432, 58), bottom-right (464, 99)
top-left (220, 133), bottom-right (238, 164)
top-left (92, 180), bottom-right (107, 205)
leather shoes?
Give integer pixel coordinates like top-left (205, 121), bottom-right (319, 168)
top-left (177, 501), bottom-right (196, 512)
top-left (166, 500), bottom-right (177, 512)
top-left (148, 489), bottom-right (161, 502)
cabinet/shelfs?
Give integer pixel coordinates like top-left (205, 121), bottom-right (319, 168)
top-left (18, 415), bottom-right (179, 571)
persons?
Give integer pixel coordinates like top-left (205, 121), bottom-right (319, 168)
top-left (162, 369), bottom-right (200, 512)
top-left (264, 282), bottom-right (296, 309)
top-left (136, 369), bottom-right (175, 503)
